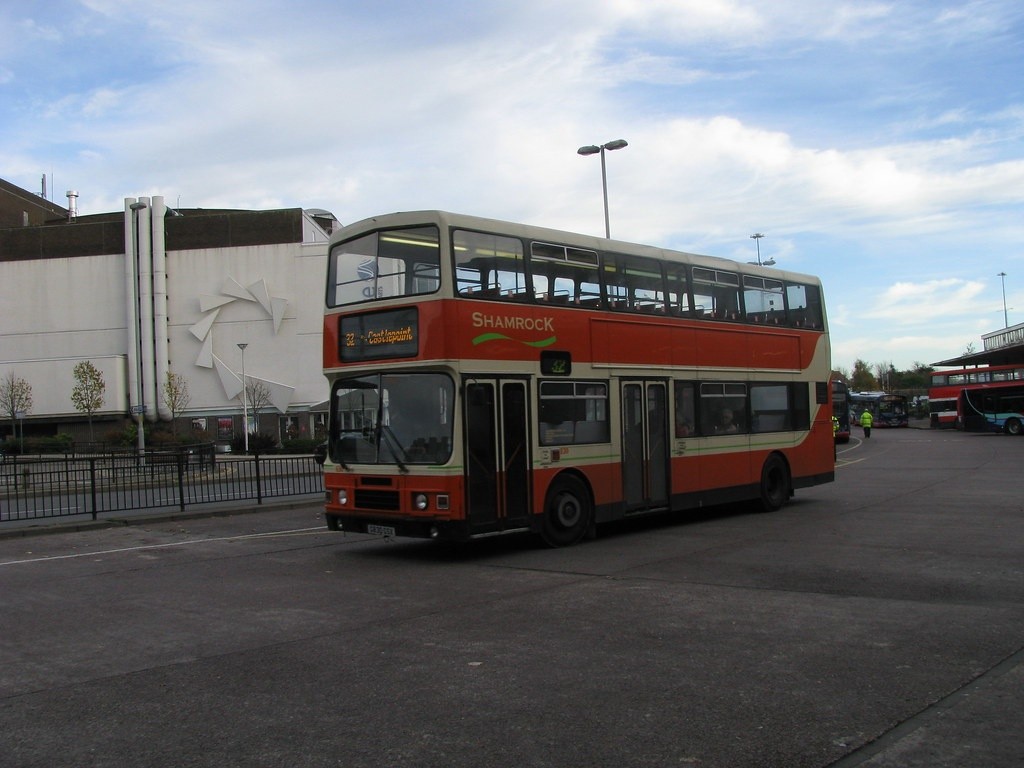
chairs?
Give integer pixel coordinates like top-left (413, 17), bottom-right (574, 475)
top-left (459, 283), bottom-right (809, 328)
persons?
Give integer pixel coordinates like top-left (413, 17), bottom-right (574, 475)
top-left (851, 410), bottom-right (858, 426)
top-left (860, 409), bottom-right (873, 438)
top-left (717, 409), bottom-right (736, 432)
top-left (832, 409), bottom-right (839, 461)
top-left (676, 391), bottom-right (694, 434)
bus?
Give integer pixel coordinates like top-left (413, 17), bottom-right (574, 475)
top-left (320, 209), bottom-right (839, 550)
top-left (957, 379), bottom-right (1024, 435)
top-left (928, 364), bottom-right (1024, 429)
top-left (849, 389), bottom-right (912, 428)
top-left (833, 380), bottom-right (852, 444)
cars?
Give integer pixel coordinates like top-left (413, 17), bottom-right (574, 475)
top-left (314, 432), bottom-right (375, 465)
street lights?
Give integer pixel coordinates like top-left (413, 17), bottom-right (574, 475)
top-left (576, 139), bottom-right (628, 306)
top-left (237, 343), bottom-right (250, 454)
top-left (749, 234), bottom-right (766, 266)
top-left (997, 271), bottom-right (1009, 329)
